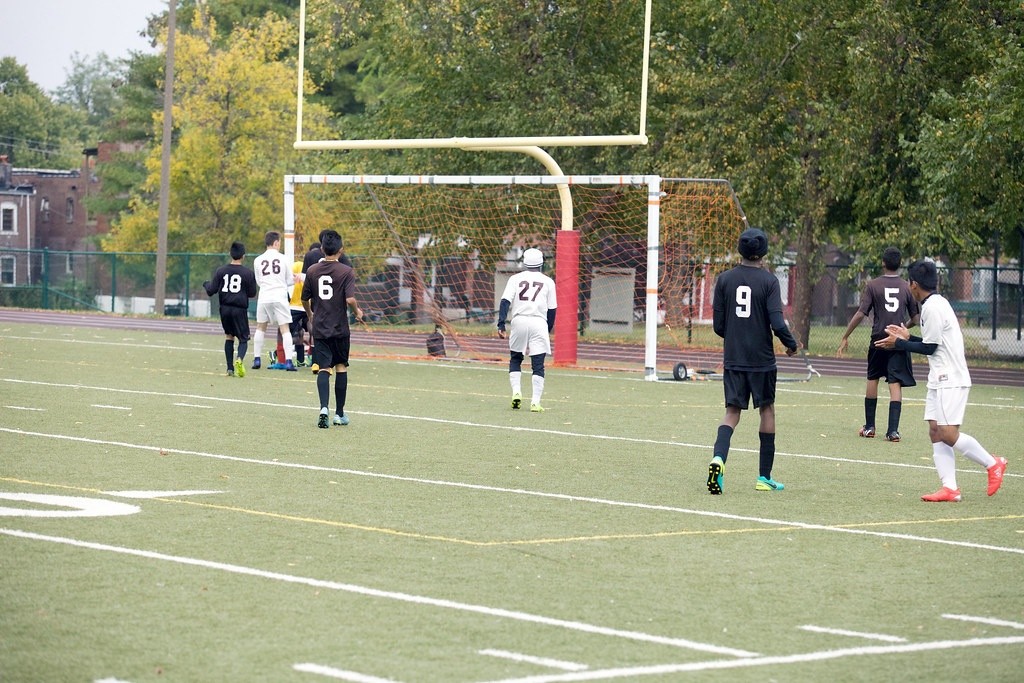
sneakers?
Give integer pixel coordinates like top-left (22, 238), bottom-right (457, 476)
top-left (226, 370), bottom-right (234, 377)
top-left (921, 487), bottom-right (962, 501)
top-left (707, 456), bottom-right (725, 494)
top-left (318, 406), bottom-right (330, 428)
top-left (511, 394), bottom-right (522, 409)
top-left (987, 454), bottom-right (1009, 495)
top-left (886, 432), bottom-right (901, 441)
top-left (333, 414), bottom-right (350, 425)
top-left (530, 404), bottom-right (544, 411)
top-left (252, 357), bottom-right (261, 369)
top-left (235, 356), bottom-right (246, 377)
top-left (267, 349), bottom-right (332, 375)
top-left (860, 425), bottom-right (875, 437)
top-left (756, 476), bottom-right (785, 491)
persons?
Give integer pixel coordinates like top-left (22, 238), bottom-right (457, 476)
top-left (835, 248), bottom-right (921, 444)
top-left (497, 247), bottom-right (557, 412)
top-left (265, 260), bottom-right (309, 367)
top-left (707, 228), bottom-right (797, 495)
top-left (202, 242), bottom-right (256, 377)
top-left (874, 259), bottom-right (1007, 503)
top-left (252, 231), bottom-right (298, 372)
top-left (300, 228), bottom-right (363, 429)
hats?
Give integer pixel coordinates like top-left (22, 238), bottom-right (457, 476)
top-left (738, 229), bottom-right (768, 260)
top-left (524, 249), bottom-right (544, 267)
